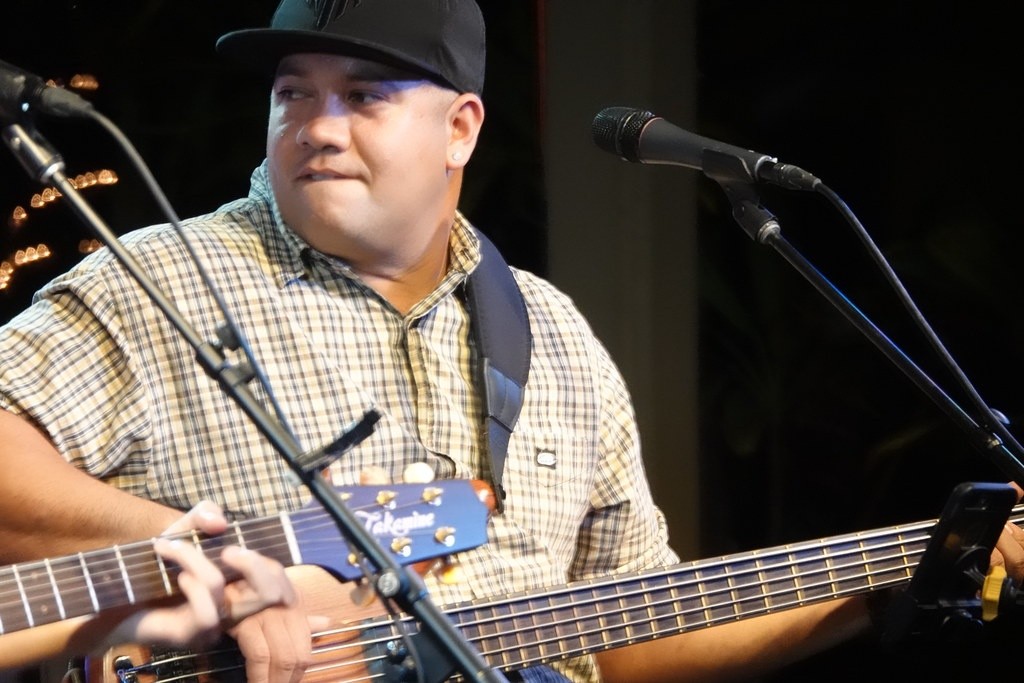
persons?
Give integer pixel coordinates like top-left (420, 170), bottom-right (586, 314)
top-left (1, 1), bottom-right (1022, 683)
top-left (0, 499), bottom-right (292, 680)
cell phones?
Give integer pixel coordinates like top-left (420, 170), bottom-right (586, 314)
top-left (887, 483), bottom-right (1017, 638)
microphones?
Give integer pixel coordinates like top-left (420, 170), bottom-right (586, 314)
top-left (592, 106), bottom-right (821, 192)
top-left (0, 60), bottom-right (90, 119)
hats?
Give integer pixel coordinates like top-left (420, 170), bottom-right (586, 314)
top-left (215, 0), bottom-right (487, 100)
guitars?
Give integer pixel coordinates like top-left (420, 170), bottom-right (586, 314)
top-left (76, 478), bottom-right (939, 681)
top-left (0, 460), bottom-right (490, 642)
